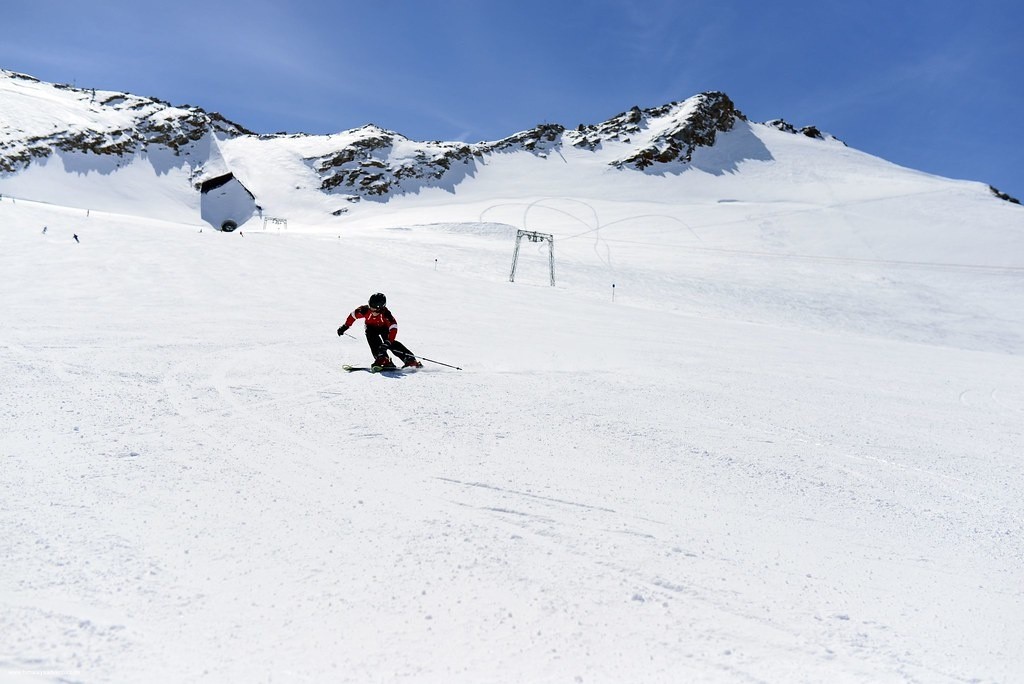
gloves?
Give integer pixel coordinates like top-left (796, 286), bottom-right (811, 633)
top-left (337, 325), bottom-right (349, 336)
top-left (379, 339), bottom-right (391, 350)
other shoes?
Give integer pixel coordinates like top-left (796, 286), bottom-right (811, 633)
top-left (371, 355), bottom-right (390, 366)
top-left (406, 360), bottom-right (418, 367)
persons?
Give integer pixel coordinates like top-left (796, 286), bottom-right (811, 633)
top-left (337, 293), bottom-right (423, 369)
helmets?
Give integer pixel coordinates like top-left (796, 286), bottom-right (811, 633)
top-left (367, 292), bottom-right (386, 312)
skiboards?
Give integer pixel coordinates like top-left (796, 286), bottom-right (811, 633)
top-left (343, 362), bottom-right (422, 373)
